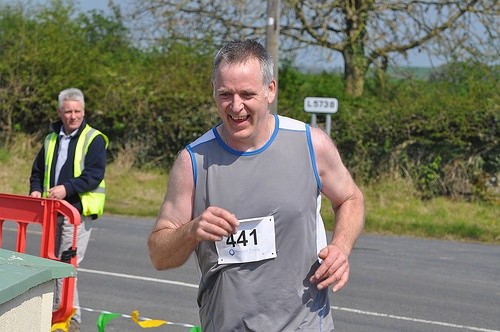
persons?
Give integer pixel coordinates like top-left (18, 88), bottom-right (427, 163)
top-left (28, 88), bottom-right (110, 332)
top-left (147, 37), bottom-right (365, 332)
